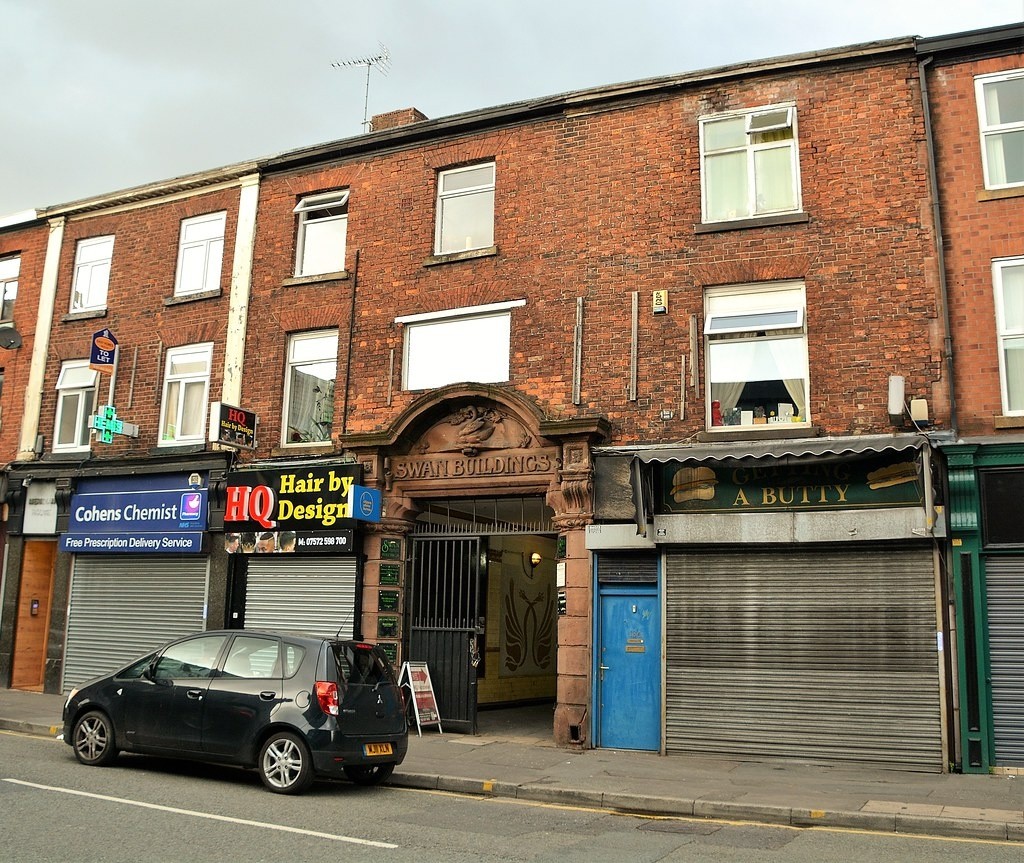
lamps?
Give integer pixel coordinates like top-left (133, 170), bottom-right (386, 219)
top-left (529, 551), bottom-right (543, 569)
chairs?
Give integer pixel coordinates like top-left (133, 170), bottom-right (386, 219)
top-left (224, 652), bottom-right (254, 676)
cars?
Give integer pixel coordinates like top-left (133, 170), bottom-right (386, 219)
top-left (60, 629), bottom-right (409, 794)
